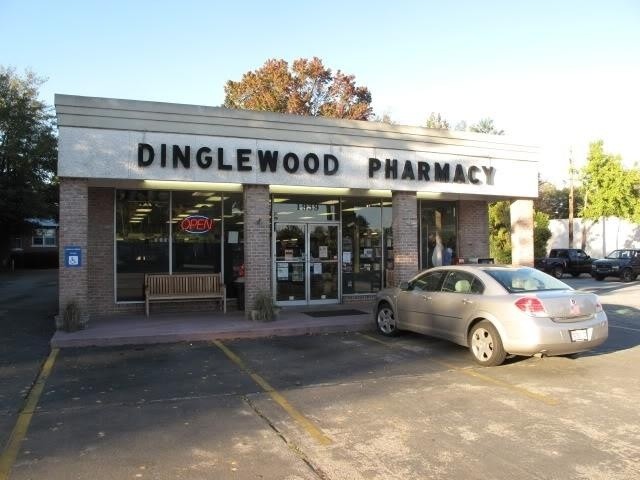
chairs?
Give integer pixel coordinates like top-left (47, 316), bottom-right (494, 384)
top-left (454, 279), bottom-right (472, 294)
top-left (522, 279), bottom-right (544, 291)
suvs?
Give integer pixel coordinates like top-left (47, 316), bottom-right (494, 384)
top-left (592, 248), bottom-right (640, 283)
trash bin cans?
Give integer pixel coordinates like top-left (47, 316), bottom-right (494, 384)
top-left (234, 277), bottom-right (245, 310)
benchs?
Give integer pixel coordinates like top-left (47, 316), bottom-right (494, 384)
top-left (144, 272), bottom-right (228, 317)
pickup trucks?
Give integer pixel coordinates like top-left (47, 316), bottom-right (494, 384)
top-left (535, 248), bottom-right (599, 279)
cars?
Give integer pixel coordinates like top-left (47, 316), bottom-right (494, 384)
top-left (375, 261), bottom-right (610, 368)
